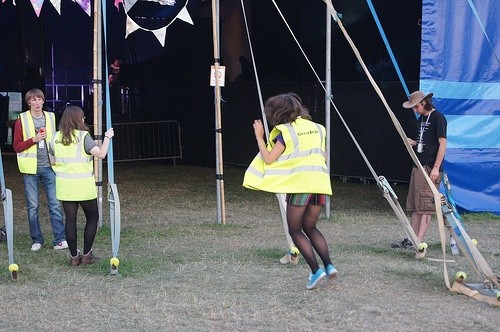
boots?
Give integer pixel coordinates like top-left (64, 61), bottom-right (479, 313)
top-left (70, 249), bottom-right (81, 265)
top-left (82, 251), bottom-right (100, 265)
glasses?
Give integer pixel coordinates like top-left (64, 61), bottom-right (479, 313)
top-left (83, 116), bottom-right (86, 119)
top-left (414, 104), bottom-right (423, 108)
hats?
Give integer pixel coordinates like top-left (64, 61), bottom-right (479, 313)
top-left (402, 91), bottom-right (433, 108)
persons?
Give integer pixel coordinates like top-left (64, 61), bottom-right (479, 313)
top-left (14, 88), bottom-right (68, 251)
top-left (48, 106), bottom-right (114, 267)
top-left (242, 93), bottom-right (337, 290)
top-left (390, 91), bottom-right (446, 249)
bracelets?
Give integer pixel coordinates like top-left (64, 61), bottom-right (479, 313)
top-left (104, 136), bottom-right (110, 139)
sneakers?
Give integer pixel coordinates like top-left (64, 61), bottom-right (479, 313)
top-left (306, 268), bottom-right (326, 289)
top-left (326, 264), bottom-right (338, 279)
top-left (390, 237), bottom-right (408, 248)
top-left (31, 244), bottom-right (41, 250)
top-left (404, 241), bottom-right (414, 248)
top-left (54, 241), bottom-right (68, 249)
